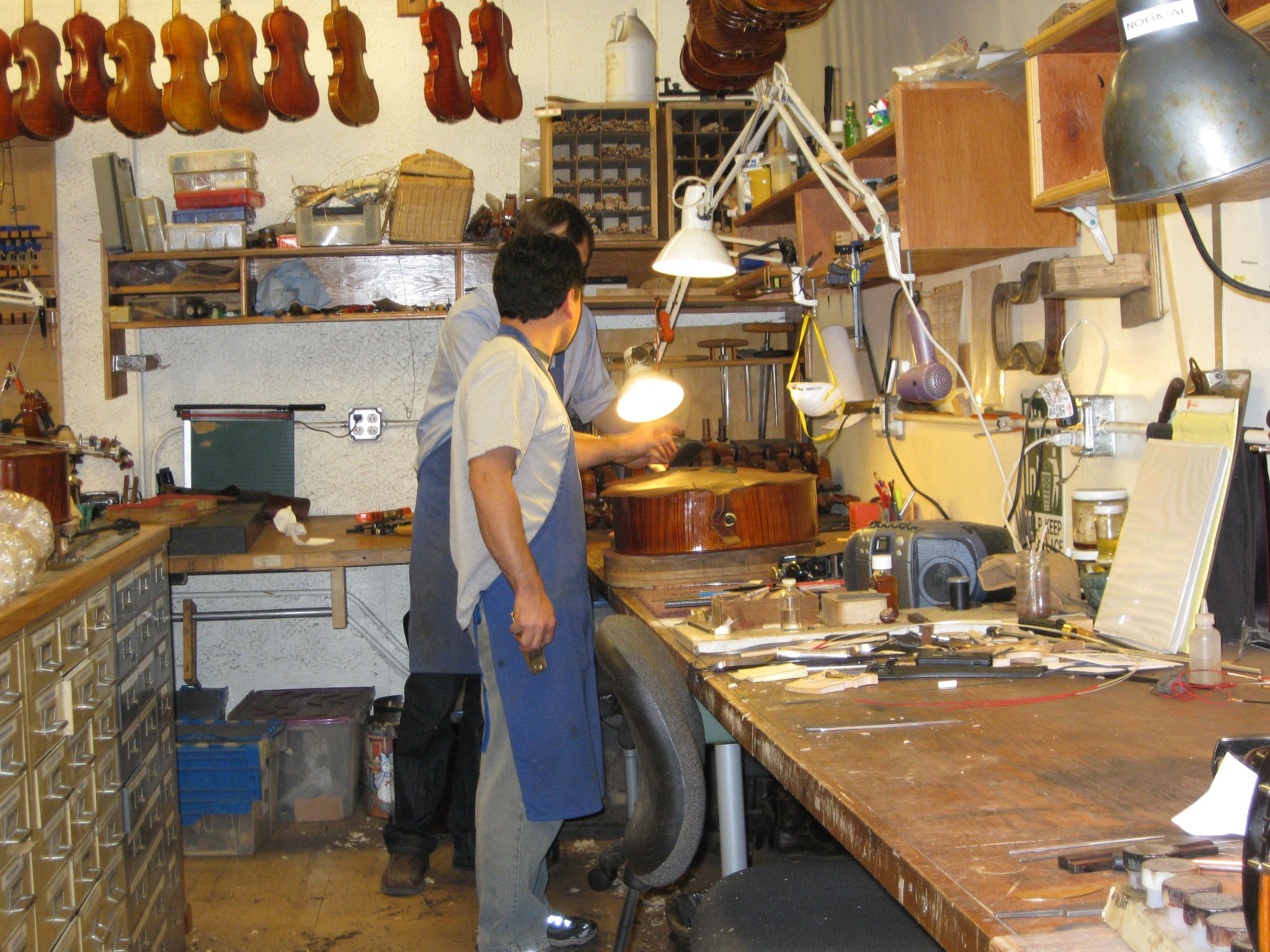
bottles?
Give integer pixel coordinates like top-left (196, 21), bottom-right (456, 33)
top-left (1072, 487), bottom-right (1128, 560)
top-left (843, 101), bottom-right (861, 151)
top-left (868, 554), bottom-right (899, 612)
top-left (605, 8), bottom-right (658, 105)
top-left (1189, 598), bottom-right (1222, 687)
top-left (796, 135), bottom-right (817, 180)
top-left (1015, 551), bottom-right (1051, 620)
top-left (770, 134), bottom-right (792, 195)
top-left (779, 586), bottom-right (801, 633)
top-left (828, 119), bottom-right (844, 151)
top-left (1092, 560), bottom-right (1112, 573)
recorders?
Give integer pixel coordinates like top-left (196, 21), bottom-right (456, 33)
top-left (843, 520), bottom-right (1015, 610)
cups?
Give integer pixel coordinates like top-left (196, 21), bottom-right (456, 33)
top-left (741, 168), bottom-right (772, 209)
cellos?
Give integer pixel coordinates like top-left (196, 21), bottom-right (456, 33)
top-left (0, 433), bottom-right (139, 528)
top-left (600, 465), bottom-right (820, 555)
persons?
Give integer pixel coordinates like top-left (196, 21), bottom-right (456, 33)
top-left (447, 233), bottom-right (684, 951)
top-left (381, 197), bottom-right (678, 897)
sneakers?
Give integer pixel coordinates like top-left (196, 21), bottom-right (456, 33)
top-left (546, 909), bottom-right (599, 946)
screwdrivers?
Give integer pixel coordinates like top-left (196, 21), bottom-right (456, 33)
top-left (39, 306), bottom-right (46, 349)
top-left (46, 290), bottom-right (57, 367)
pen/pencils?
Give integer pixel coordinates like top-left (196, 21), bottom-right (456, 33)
top-left (722, 584), bottom-right (777, 592)
top-left (875, 474), bottom-right (916, 523)
top-left (699, 590), bottom-right (722, 596)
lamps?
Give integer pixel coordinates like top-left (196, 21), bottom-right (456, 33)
top-left (1102, 0), bottom-right (1270, 201)
top-left (653, 62), bottom-right (917, 282)
top-left (614, 235), bottom-right (781, 423)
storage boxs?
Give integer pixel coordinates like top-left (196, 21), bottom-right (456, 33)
top-left (175, 720), bottom-right (287, 857)
top-left (110, 306), bottom-right (133, 323)
top-left (92, 150), bottom-right (266, 253)
top-left (167, 503), bottom-right (265, 555)
top-left (711, 589), bottom-right (819, 629)
top-left (296, 206), bottom-right (382, 246)
top-left (227, 686), bottom-right (375, 822)
top-left (582, 276), bottom-right (628, 297)
top-left (132, 296), bottom-right (204, 321)
top-left (821, 591), bottom-right (886, 627)
top-left (176, 686), bottom-right (229, 724)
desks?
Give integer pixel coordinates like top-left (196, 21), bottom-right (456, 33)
top-left (581, 529), bottom-right (1270, 952)
top-left (168, 514), bottom-right (412, 684)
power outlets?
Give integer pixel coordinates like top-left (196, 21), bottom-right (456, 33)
top-left (872, 396), bottom-right (904, 440)
top-left (1071, 394), bottom-right (1116, 456)
top-left (349, 408), bottom-right (382, 440)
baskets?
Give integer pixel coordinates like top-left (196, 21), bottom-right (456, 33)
top-left (388, 174), bottom-right (472, 244)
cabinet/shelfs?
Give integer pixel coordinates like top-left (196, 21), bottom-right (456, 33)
top-left (732, 79), bottom-right (1083, 296)
top-left (540, 101), bottom-right (775, 240)
top-left (1023, 0), bottom-right (1270, 208)
top-left (2, 543), bottom-right (187, 952)
top-left (101, 241), bottom-right (794, 399)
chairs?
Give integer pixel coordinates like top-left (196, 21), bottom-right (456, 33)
top-left (589, 614), bottom-right (946, 952)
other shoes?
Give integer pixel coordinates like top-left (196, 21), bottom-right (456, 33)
top-left (452, 846), bottom-right (476, 869)
top-left (380, 848), bottom-right (430, 896)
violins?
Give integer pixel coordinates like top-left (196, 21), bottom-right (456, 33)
top-left (160, 0), bottom-right (219, 137)
top-left (61, 0), bottom-right (115, 122)
top-left (580, 440), bottom-right (861, 530)
top-left (679, 0), bottom-right (835, 92)
top-left (0, 29), bottom-right (19, 144)
top-left (323, 0), bottom-right (380, 129)
top-left (418, 0), bottom-right (474, 125)
top-left (208, 0), bottom-right (269, 133)
top-left (468, 0), bottom-right (523, 126)
top-left (11, 0), bottom-right (75, 142)
top-left (262, 0), bottom-right (319, 122)
top-left (105, 0), bottom-right (168, 140)
